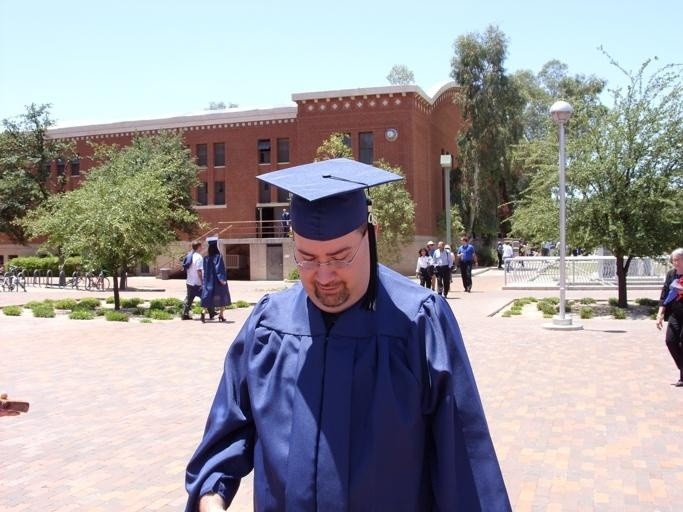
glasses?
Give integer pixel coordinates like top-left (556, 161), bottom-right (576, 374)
top-left (293, 227), bottom-right (369, 272)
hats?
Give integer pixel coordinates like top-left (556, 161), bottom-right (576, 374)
top-left (427, 241), bottom-right (435, 245)
top-left (206, 237), bottom-right (218, 244)
top-left (253, 155), bottom-right (406, 241)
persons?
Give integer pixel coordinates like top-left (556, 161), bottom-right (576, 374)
top-left (280, 208), bottom-right (291, 237)
top-left (200, 237), bottom-right (231, 323)
top-left (415, 240), bottom-right (455, 298)
top-left (455, 237), bottom-right (479, 292)
top-left (184, 158), bottom-right (511, 510)
top-left (182, 241), bottom-right (219, 320)
top-left (656, 248), bottom-right (683, 387)
top-left (497, 239), bottom-right (581, 268)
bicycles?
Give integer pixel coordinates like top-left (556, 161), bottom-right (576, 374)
top-left (85, 271), bottom-right (110, 291)
top-left (62, 273), bottom-right (84, 287)
top-left (0, 265), bottom-right (26, 291)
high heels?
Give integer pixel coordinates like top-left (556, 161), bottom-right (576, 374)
top-left (218, 315), bottom-right (226, 322)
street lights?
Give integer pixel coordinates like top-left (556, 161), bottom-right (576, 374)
top-left (550, 101), bottom-right (573, 317)
top-left (440, 154), bottom-right (453, 246)
